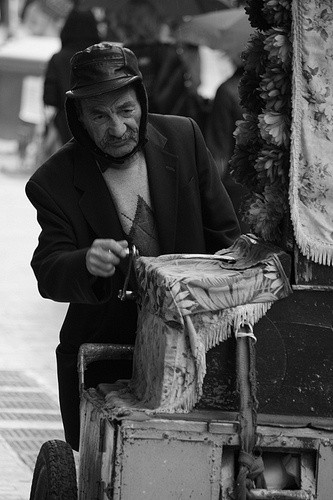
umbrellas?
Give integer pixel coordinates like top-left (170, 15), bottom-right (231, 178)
top-left (170, 8), bottom-right (252, 64)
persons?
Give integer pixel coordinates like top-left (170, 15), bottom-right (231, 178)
top-left (16, 0), bottom-right (258, 218)
top-left (25, 41), bottom-right (240, 454)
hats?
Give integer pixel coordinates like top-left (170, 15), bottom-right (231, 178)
top-left (64, 41), bottom-right (148, 160)
